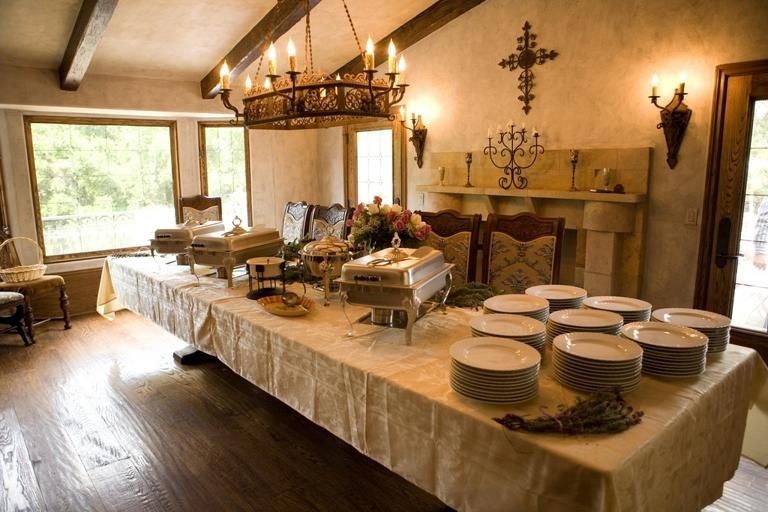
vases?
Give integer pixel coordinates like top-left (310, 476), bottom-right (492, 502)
top-left (367, 240), bottom-right (395, 324)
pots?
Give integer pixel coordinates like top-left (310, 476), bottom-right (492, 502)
top-left (298, 232), bottom-right (361, 279)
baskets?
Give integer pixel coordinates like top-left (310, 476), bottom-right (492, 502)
top-left (0, 237), bottom-right (47, 283)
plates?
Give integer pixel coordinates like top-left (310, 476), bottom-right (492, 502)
top-left (259, 295), bottom-right (314, 317)
top-left (446, 282), bottom-right (733, 406)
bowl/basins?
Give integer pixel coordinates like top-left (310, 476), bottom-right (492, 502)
top-left (245, 256), bottom-right (285, 278)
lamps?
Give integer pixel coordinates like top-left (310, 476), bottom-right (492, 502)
top-left (218, 1), bottom-right (411, 134)
top-left (396, 103), bottom-right (427, 169)
top-left (648, 70), bottom-right (693, 170)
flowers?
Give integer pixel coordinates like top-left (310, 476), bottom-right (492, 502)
top-left (346, 195), bottom-right (426, 321)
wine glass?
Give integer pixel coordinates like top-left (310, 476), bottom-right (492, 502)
top-left (601, 168), bottom-right (614, 192)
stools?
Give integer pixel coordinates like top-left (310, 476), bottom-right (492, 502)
top-left (0, 292), bottom-right (31, 350)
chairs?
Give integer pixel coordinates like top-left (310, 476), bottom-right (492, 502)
top-left (410, 208), bottom-right (479, 285)
top-left (310, 203), bottom-right (355, 240)
top-left (281, 201), bottom-right (313, 243)
top-left (481, 211), bottom-right (565, 294)
top-left (1, 223), bottom-right (72, 340)
top-left (179, 194), bottom-right (223, 224)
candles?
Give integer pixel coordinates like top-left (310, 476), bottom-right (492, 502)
top-left (487, 119), bottom-right (540, 140)
